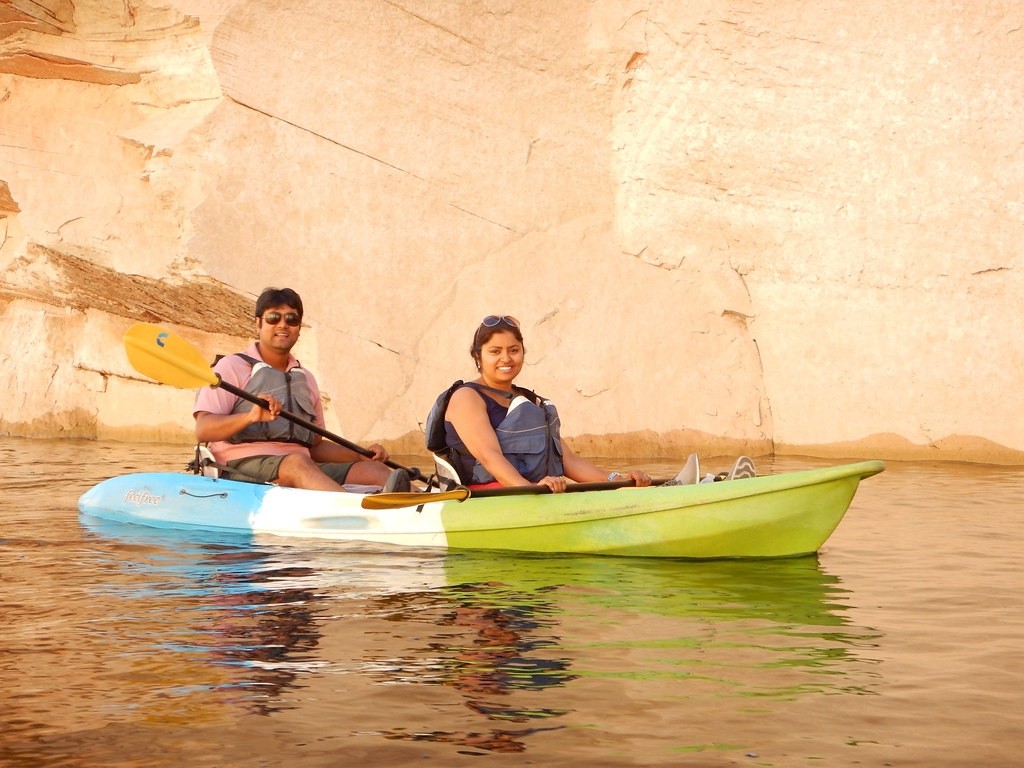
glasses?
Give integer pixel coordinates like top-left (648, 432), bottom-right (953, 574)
top-left (262, 311), bottom-right (301, 326)
top-left (475, 315), bottom-right (521, 339)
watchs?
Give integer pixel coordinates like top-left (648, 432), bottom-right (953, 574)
top-left (607, 471), bottom-right (621, 482)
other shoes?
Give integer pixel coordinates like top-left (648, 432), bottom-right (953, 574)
top-left (381, 467), bottom-right (412, 493)
top-left (671, 452), bottom-right (700, 486)
top-left (723, 455), bottom-right (756, 479)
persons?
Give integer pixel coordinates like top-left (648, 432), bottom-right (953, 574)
top-left (445, 315), bottom-right (757, 493)
top-left (192, 286), bottom-right (423, 495)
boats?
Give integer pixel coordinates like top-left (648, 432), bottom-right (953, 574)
top-left (75, 458), bottom-right (888, 561)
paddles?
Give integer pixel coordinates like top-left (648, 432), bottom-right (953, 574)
top-left (123, 319), bottom-right (441, 489)
top-left (360, 475), bottom-right (728, 511)
